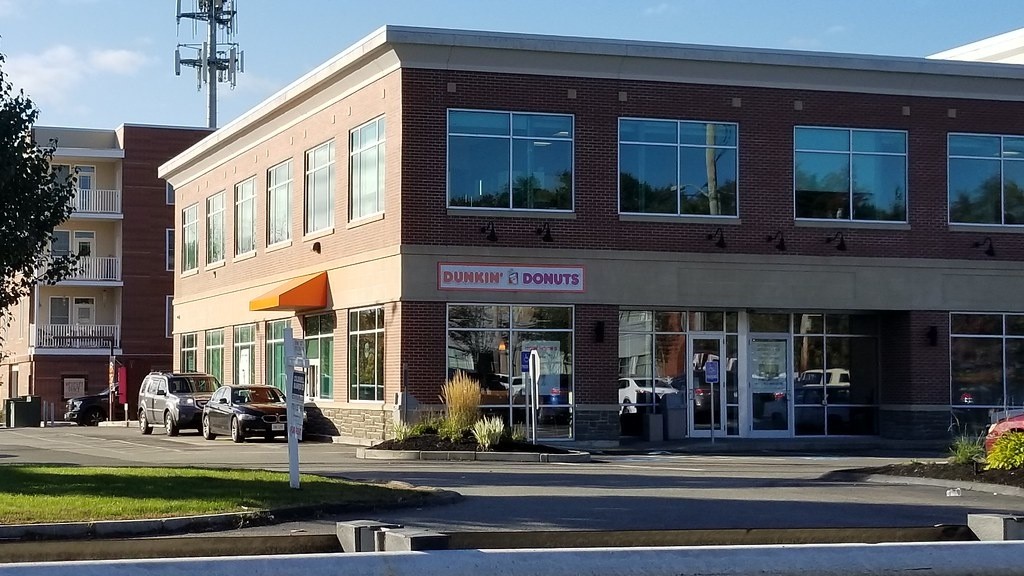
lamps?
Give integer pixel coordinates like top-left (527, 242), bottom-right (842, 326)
top-left (708, 227), bottom-right (728, 248)
top-left (972, 237), bottom-right (995, 256)
top-left (826, 232), bottom-right (847, 250)
top-left (481, 221), bottom-right (498, 242)
top-left (926, 325), bottom-right (938, 347)
top-left (768, 231), bottom-right (787, 250)
top-left (536, 222), bottom-right (553, 242)
top-left (593, 321), bottom-right (605, 343)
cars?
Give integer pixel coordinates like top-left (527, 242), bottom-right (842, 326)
top-left (672, 378), bottom-right (731, 410)
top-left (201, 384), bottom-right (308, 443)
top-left (620, 378), bottom-right (679, 413)
top-left (954, 384), bottom-right (995, 421)
top-left (986, 413), bottom-right (1024, 456)
top-left (752, 368), bottom-right (850, 403)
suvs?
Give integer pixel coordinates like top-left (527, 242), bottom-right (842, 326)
top-left (63, 382), bottom-right (137, 426)
top-left (137, 369), bottom-right (224, 437)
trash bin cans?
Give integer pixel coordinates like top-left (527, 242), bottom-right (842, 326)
top-left (659, 392), bottom-right (687, 439)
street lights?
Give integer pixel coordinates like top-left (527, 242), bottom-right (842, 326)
top-left (672, 183), bottom-right (722, 216)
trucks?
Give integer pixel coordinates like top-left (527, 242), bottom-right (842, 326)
top-left (511, 374), bottom-right (570, 420)
top-left (494, 374), bottom-right (509, 390)
